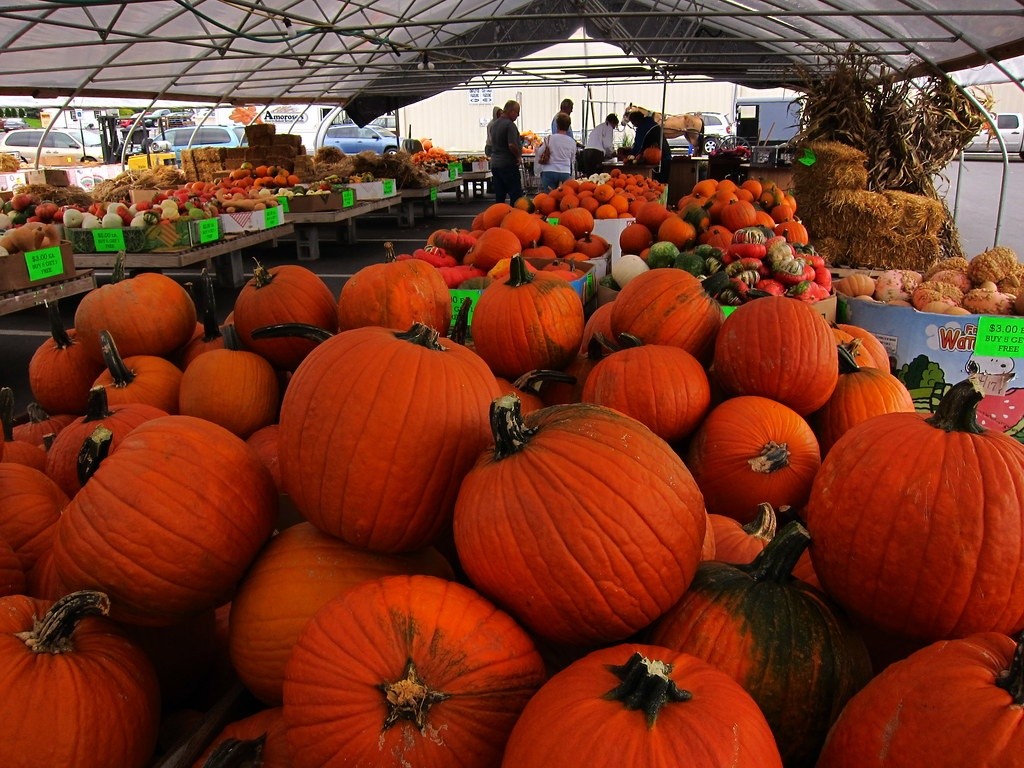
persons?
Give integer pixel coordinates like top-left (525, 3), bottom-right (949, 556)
top-left (485, 100), bottom-right (522, 206)
top-left (539, 113), bottom-right (576, 194)
top-left (552, 98), bottom-right (585, 148)
top-left (630, 112), bottom-right (672, 184)
top-left (581, 114), bottom-right (618, 176)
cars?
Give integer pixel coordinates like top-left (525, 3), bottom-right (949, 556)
top-left (129, 108), bottom-right (217, 128)
top-left (0, 117), bottom-right (7, 129)
top-left (370, 115), bottom-right (394, 134)
top-left (3, 118), bottom-right (30, 133)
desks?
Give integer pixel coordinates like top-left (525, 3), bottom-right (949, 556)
top-left (602, 155), bottom-right (794, 204)
top-left (73, 223), bottom-right (296, 294)
top-left (520, 154), bottom-right (540, 192)
top-left (0, 266), bottom-right (96, 315)
top-left (282, 172), bottom-right (490, 261)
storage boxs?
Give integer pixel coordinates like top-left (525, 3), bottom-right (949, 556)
top-left (446, 183), bottom-right (1024, 446)
top-left (0, 152), bottom-right (177, 193)
top-left (0, 242), bottom-right (76, 292)
top-left (64, 160), bottom-right (490, 253)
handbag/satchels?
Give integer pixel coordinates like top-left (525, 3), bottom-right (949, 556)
top-left (537, 135), bottom-right (550, 164)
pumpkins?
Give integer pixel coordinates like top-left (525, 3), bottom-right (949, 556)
top-left (0, 138), bottom-right (1024, 768)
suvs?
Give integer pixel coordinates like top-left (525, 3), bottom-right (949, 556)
top-left (140, 124), bottom-right (247, 150)
top-left (957, 113), bottom-right (1024, 159)
top-left (0, 129), bottom-right (106, 166)
top-left (659, 111), bottom-right (734, 156)
top-left (324, 124), bottom-right (398, 157)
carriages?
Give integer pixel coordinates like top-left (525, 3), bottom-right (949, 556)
top-left (621, 95), bottom-right (803, 163)
